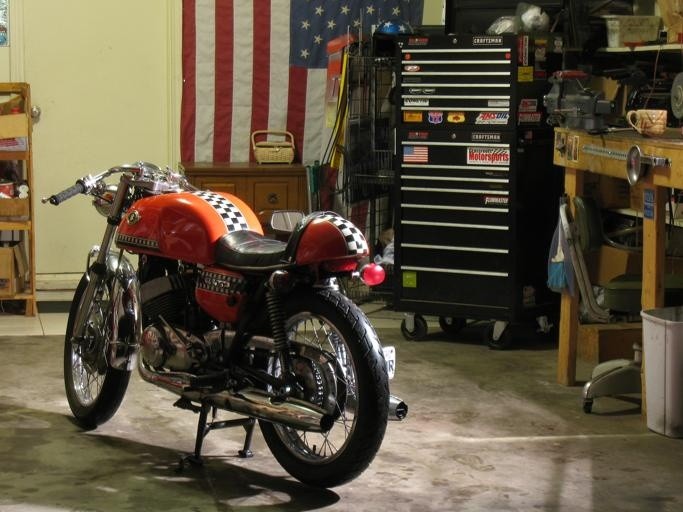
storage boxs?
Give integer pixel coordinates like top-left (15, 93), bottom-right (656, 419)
top-left (602, 15), bottom-right (661, 48)
top-left (586, 243), bottom-right (683, 288)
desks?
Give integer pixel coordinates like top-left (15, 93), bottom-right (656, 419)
top-left (553, 125), bottom-right (683, 420)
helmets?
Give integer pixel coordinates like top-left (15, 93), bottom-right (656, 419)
top-left (373, 17), bottom-right (414, 49)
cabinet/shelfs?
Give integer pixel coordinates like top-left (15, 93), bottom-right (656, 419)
top-left (181, 161), bottom-right (309, 235)
top-left (0, 82), bottom-right (37, 317)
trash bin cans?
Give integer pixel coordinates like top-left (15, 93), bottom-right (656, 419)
top-left (641, 306), bottom-right (683, 438)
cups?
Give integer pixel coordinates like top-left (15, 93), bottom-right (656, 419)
top-left (625, 109), bottom-right (668, 139)
top-left (0, 181), bottom-right (14, 197)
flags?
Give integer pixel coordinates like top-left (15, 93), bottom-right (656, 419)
top-left (178, 1), bottom-right (426, 163)
top-left (402, 146), bottom-right (430, 165)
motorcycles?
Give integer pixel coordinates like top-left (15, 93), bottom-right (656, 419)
top-left (42, 160), bottom-right (408, 488)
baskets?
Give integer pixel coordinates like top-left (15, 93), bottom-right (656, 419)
top-left (251, 130), bottom-right (295, 165)
